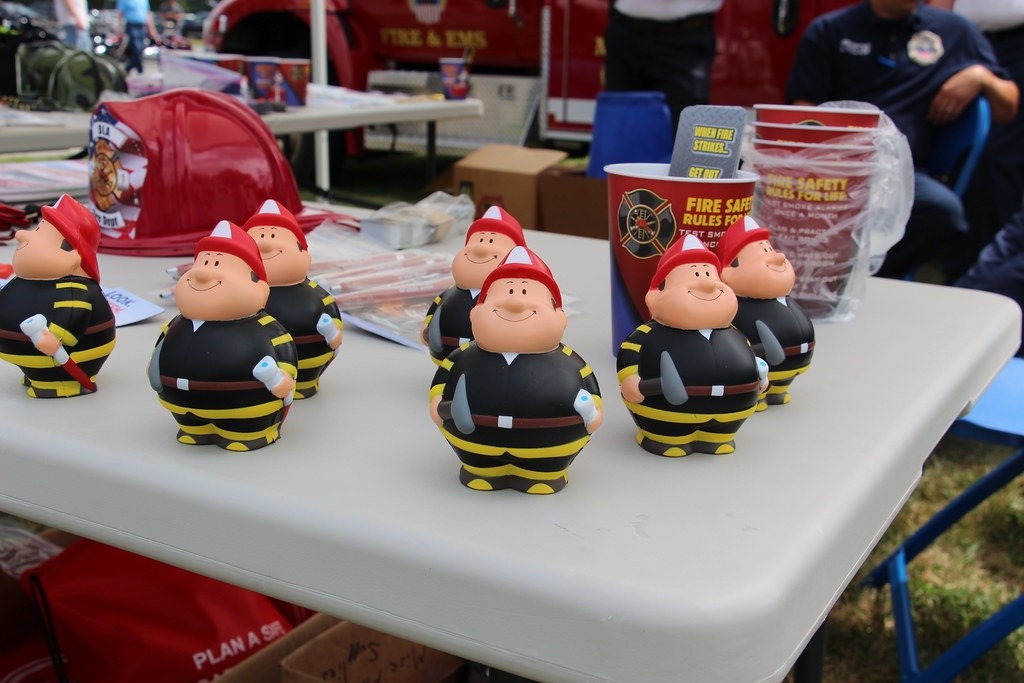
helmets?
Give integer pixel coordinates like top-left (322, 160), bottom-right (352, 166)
top-left (87, 87), bottom-right (362, 257)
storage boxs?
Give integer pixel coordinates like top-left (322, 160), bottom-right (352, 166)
top-left (540, 157), bottom-right (610, 242)
top-left (455, 143), bottom-right (568, 229)
top-left (0, 528), bottom-right (466, 683)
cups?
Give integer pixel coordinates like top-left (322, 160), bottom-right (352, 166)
top-left (163, 48), bottom-right (311, 110)
top-left (753, 103), bottom-right (883, 321)
top-left (439, 57), bottom-right (472, 100)
top-left (602, 162), bottom-right (762, 360)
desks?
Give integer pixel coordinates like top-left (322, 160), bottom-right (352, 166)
top-left (0, 85), bottom-right (484, 152)
top-left (0, 201), bottom-right (1024, 683)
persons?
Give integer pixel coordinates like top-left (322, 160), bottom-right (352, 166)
top-left (715, 214), bottom-right (816, 411)
top-left (54, 0), bottom-right (92, 53)
top-left (616, 234), bottom-right (767, 458)
top-left (429, 247), bottom-right (602, 495)
top-left (603, 0), bottom-right (724, 138)
top-left (421, 206), bottom-right (527, 369)
top-left (238, 200), bottom-right (345, 400)
top-left (785, 0), bottom-right (1024, 277)
top-left (116, 0), bottom-right (161, 72)
top-left (147, 219), bottom-right (299, 452)
top-left (0, 195), bottom-right (116, 398)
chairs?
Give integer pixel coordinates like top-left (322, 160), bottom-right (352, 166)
top-left (904, 93), bottom-right (991, 279)
top-left (855, 357), bottom-right (1024, 683)
